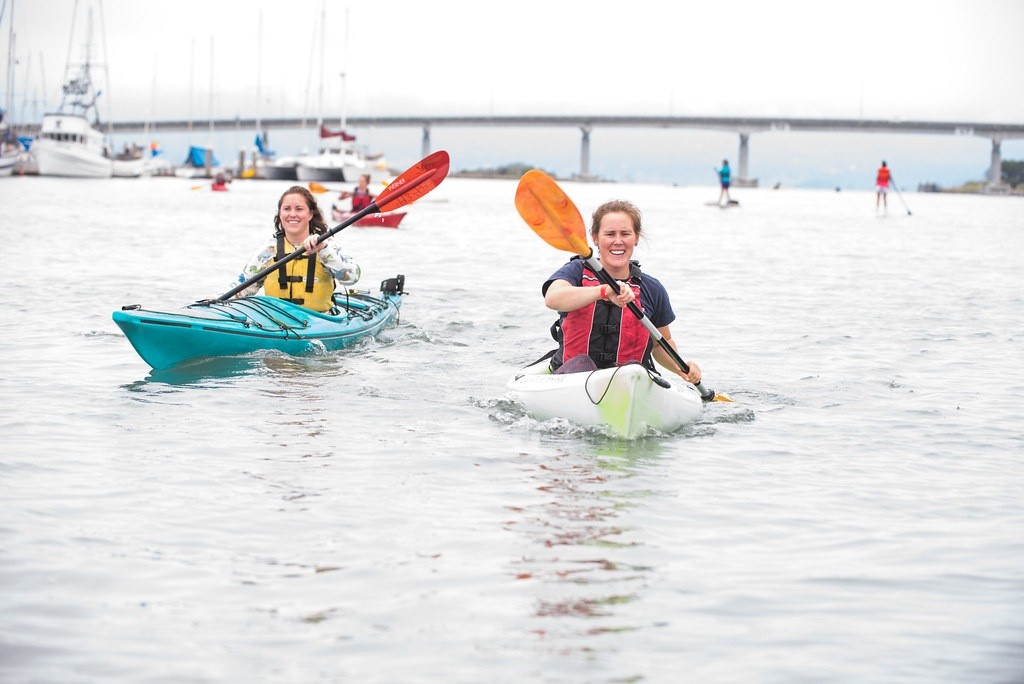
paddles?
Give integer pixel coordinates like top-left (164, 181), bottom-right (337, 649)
top-left (513, 167), bottom-right (732, 403)
top-left (889, 176), bottom-right (912, 215)
top-left (218, 150), bottom-right (450, 301)
top-left (190, 169), bottom-right (254, 191)
top-left (309, 183), bottom-right (377, 197)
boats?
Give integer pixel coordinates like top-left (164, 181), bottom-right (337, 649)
top-left (510, 359), bottom-right (703, 437)
top-left (705, 201), bottom-right (739, 208)
top-left (112, 275), bottom-right (410, 369)
top-left (332, 204), bottom-right (408, 227)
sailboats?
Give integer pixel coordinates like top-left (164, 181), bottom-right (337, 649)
top-left (1, 0), bottom-right (387, 183)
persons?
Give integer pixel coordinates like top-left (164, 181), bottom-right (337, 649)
top-left (337, 173), bottom-right (378, 213)
top-left (203, 186), bottom-right (361, 315)
top-left (715, 160), bottom-right (733, 205)
top-left (215, 172), bottom-right (229, 186)
top-left (543, 199), bottom-right (702, 386)
top-left (874, 160), bottom-right (891, 212)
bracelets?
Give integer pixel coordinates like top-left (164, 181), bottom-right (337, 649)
top-left (600, 281), bottom-right (608, 303)
